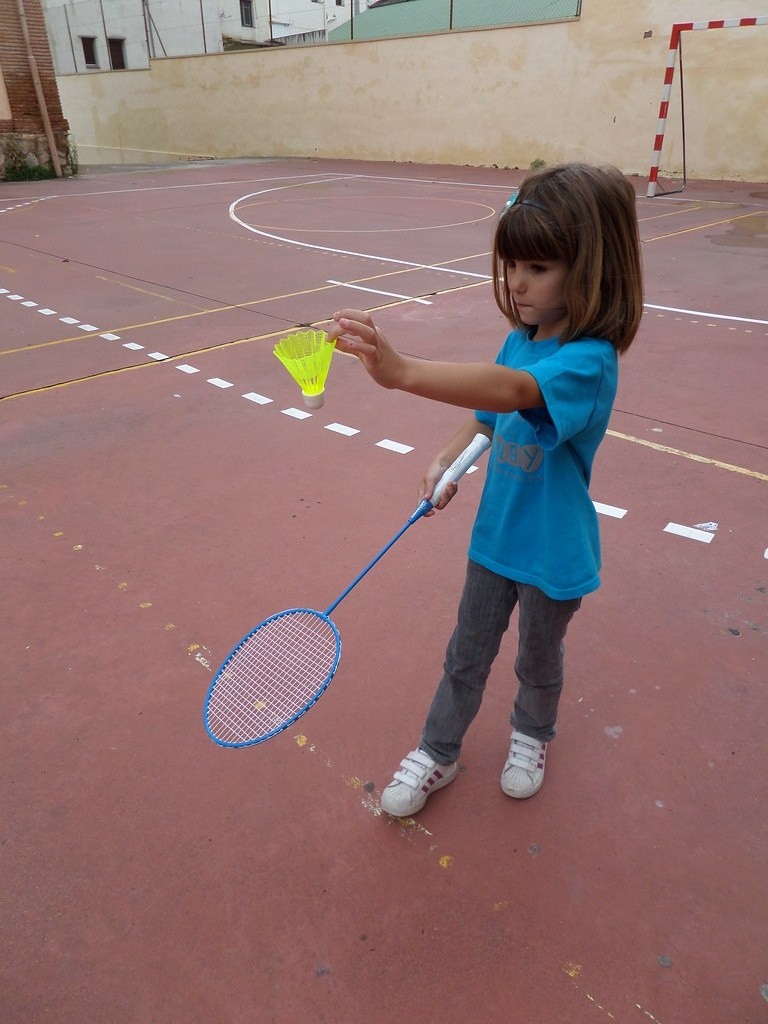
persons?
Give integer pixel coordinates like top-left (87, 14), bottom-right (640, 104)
top-left (326, 164), bottom-right (645, 817)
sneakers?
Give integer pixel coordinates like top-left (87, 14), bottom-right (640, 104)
top-left (500, 729), bottom-right (548, 799)
top-left (380, 745), bottom-right (458, 817)
